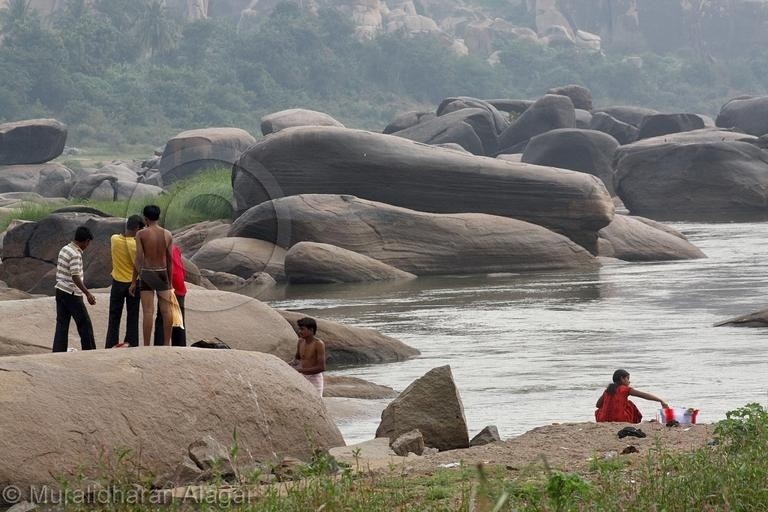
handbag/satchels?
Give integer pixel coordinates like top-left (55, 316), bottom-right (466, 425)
top-left (617, 427), bottom-right (646, 439)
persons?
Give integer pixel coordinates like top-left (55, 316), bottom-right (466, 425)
top-left (591, 368), bottom-right (669, 424)
top-left (286, 316), bottom-right (326, 400)
top-left (128, 203), bottom-right (176, 346)
top-left (103, 215), bottom-right (144, 345)
top-left (51, 224), bottom-right (98, 354)
top-left (153, 245), bottom-right (187, 347)
